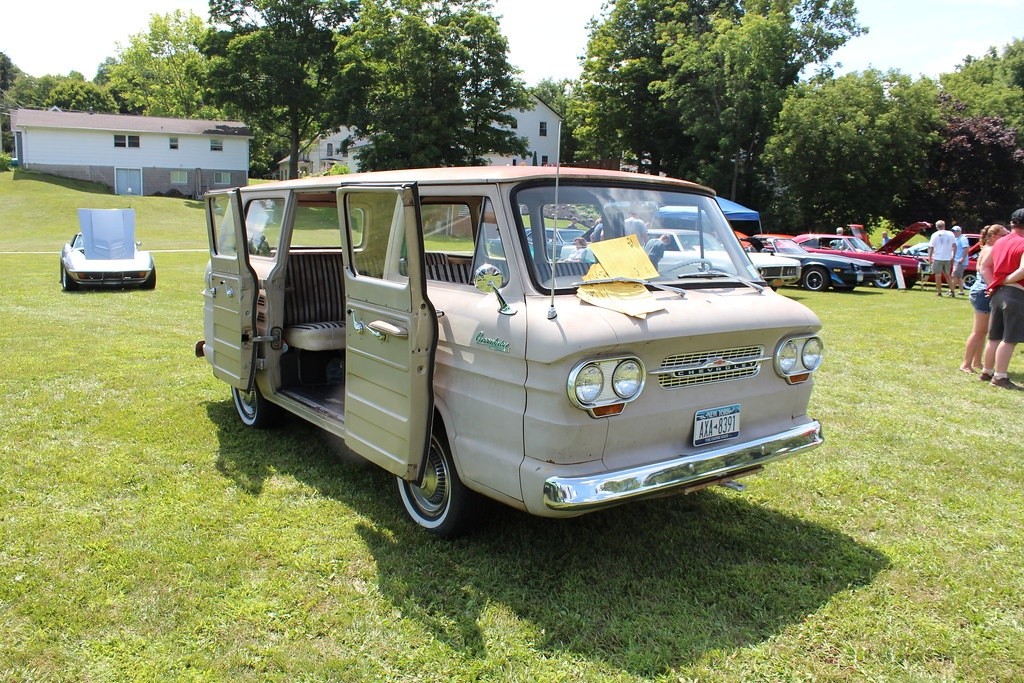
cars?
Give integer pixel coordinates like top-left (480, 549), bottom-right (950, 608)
top-left (904, 233), bottom-right (985, 290)
top-left (648, 229), bottom-right (802, 295)
top-left (792, 222), bottom-right (933, 290)
top-left (753, 237), bottom-right (880, 291)
top-left (60, 208), bottom-right (156, 291)
top-left (488, 227), bottom-right (590, 262)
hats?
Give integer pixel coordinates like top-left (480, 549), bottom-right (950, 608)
top-left (1011, 208), bottom-right (1024, 225)
top-left (950, 226), bottom-right (962, 232)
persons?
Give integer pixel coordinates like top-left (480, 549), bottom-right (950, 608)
top-left (882, 231), bottom-right (891, 247)
top-left (980, 208), bottom-right (1024, 389)
top-left (739, 231), bottom-right (768, 253)
top-left (566, 207), bottom-right (671, 272)
top-left (829, 227), bottom-right (844, 250)
top-left (959, 224), bottom-right (1011, 374)
top-left (929, 220), bottom-right (957, 297)
top-left (947, 226), bottom-right (970, 295)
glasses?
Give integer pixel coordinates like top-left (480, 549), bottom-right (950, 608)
top-left (952, 230), bottom-right (959, 233)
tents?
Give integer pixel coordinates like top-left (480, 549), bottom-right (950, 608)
top-left (602, 192), bottom-right (767, 244)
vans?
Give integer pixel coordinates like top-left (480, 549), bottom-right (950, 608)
top-left (196, 167), bottom-right (825, 542)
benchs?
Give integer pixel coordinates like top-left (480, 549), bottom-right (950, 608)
top-left (282, 252), bottom-right (449, 383)
top-left (426, 262), bottom-right (598, 288)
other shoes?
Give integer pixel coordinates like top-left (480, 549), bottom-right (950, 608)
top-left (959, 364), bottom-right (978, 375)
top-left (947, 290), bottom-right (955, 297)
top-left (937, 293), bottom-right (942, 297)
top-left (989, 375), bottom-right (1024, 392)
top-left (958, 291), bottom-right (964, 295)
top-left (980, 371), bottom-right (994, 381)
